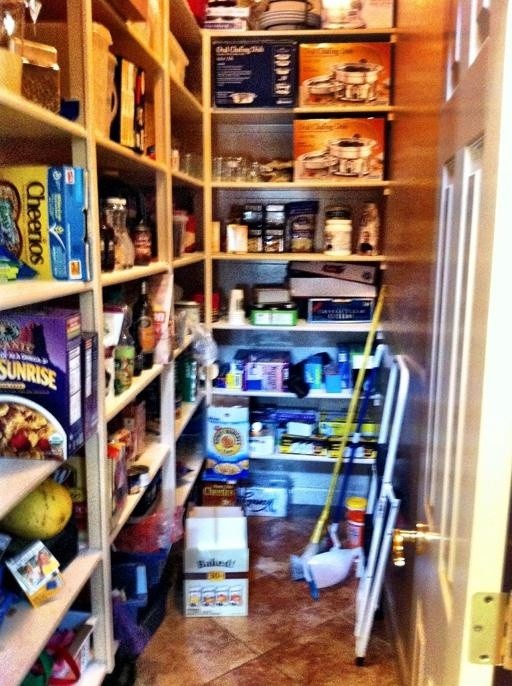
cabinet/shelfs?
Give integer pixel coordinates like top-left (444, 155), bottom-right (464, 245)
top-left (0, 0), bottom-right (207, 686)
top-left (207, 31), bottom-right (400, 505)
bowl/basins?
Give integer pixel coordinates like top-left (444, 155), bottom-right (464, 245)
top-left (230, 91), bottom-right (258, 106)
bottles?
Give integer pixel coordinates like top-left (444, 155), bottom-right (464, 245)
top-left (130, 281), bottom-right (156, 369)
top-left (321, 210), bottom-right (353, 257)
top-left (336, 343), bottom-right (350, 390)
top-left (100, 195), bottom-right (153, 273)
top-left (0, 1), bottom-right (61, 114)
top-left (242, 200), bottom-right (287, 253)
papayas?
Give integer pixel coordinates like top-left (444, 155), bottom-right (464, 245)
top-left (1, 477), bottom-right (72, 540)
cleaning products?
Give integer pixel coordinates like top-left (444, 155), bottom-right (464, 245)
top-left (304, 548), bottom-right (369, 598)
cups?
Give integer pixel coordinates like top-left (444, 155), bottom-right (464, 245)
top-left (325, 374), bottom-right (343, 394)
top-left (227, 288), bottom-right (247, 327)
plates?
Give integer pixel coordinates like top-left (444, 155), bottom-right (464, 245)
top-left (259, 9), bottom-right (321, 31)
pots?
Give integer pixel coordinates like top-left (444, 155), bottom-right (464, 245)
top-left (298, 134), bottom-right (379, 177)
top-left (305, 56), bottom-right (383, 102)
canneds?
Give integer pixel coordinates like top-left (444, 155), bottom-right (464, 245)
top-left (127, 464), bottom-right (152, 494)
top-left (323, 220), bottom-right (354, 256)
top-left (176, 299), bottom-right (201, 322)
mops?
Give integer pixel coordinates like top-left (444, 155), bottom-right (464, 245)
top-left (291, 285), bottom-right (386, 581)
